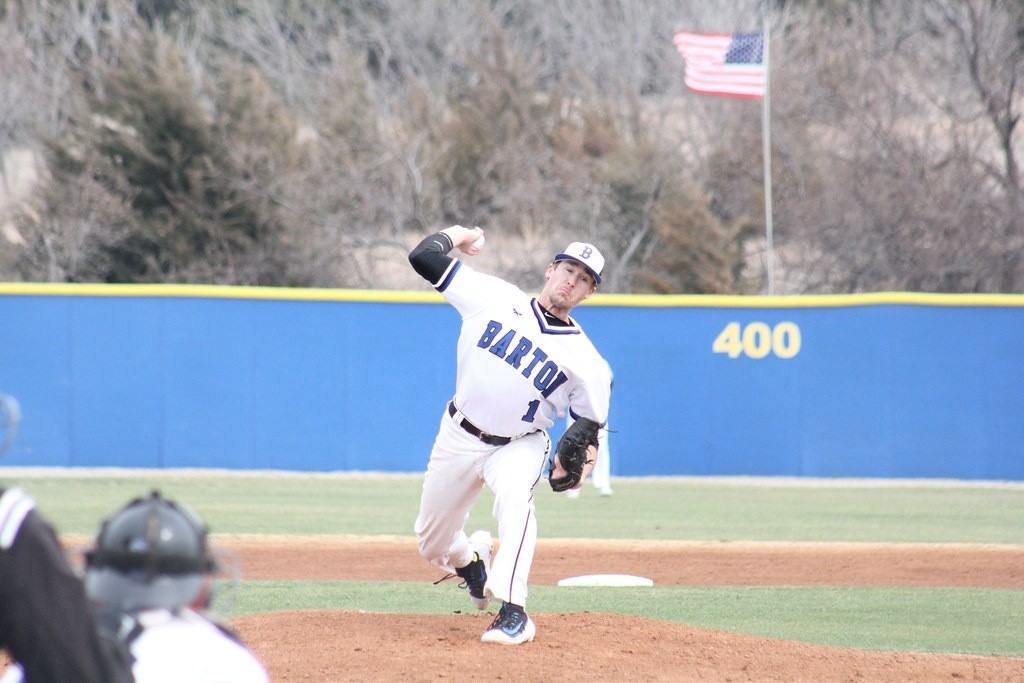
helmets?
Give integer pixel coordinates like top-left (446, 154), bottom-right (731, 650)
top-left (82, 489), bottom-right (212, 611)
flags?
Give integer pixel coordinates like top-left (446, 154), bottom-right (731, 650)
top-left (674, 32), bottom-right (766, 100)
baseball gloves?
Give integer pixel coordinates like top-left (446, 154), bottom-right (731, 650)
top-left (546, 416), bottom-right (601, 493)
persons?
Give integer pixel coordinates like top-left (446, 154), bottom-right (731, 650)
top-left (408, 225), bottom-right (614, 645)
top-left (0, 485), bottom-right (269, 683)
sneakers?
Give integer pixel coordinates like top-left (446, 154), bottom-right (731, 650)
top-left (454, 530), bottom-right (495, 610)
top-left (480, 603), bottom-right (536, 645)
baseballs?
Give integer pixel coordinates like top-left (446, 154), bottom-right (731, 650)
top-left (470, 229), bottom-right (485, 251)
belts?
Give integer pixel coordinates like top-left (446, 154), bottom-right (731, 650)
top-left (448, 400), bottom-right (521, 446)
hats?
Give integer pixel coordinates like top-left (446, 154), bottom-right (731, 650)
top-left (553, 242), bottom-right (606, 287)
